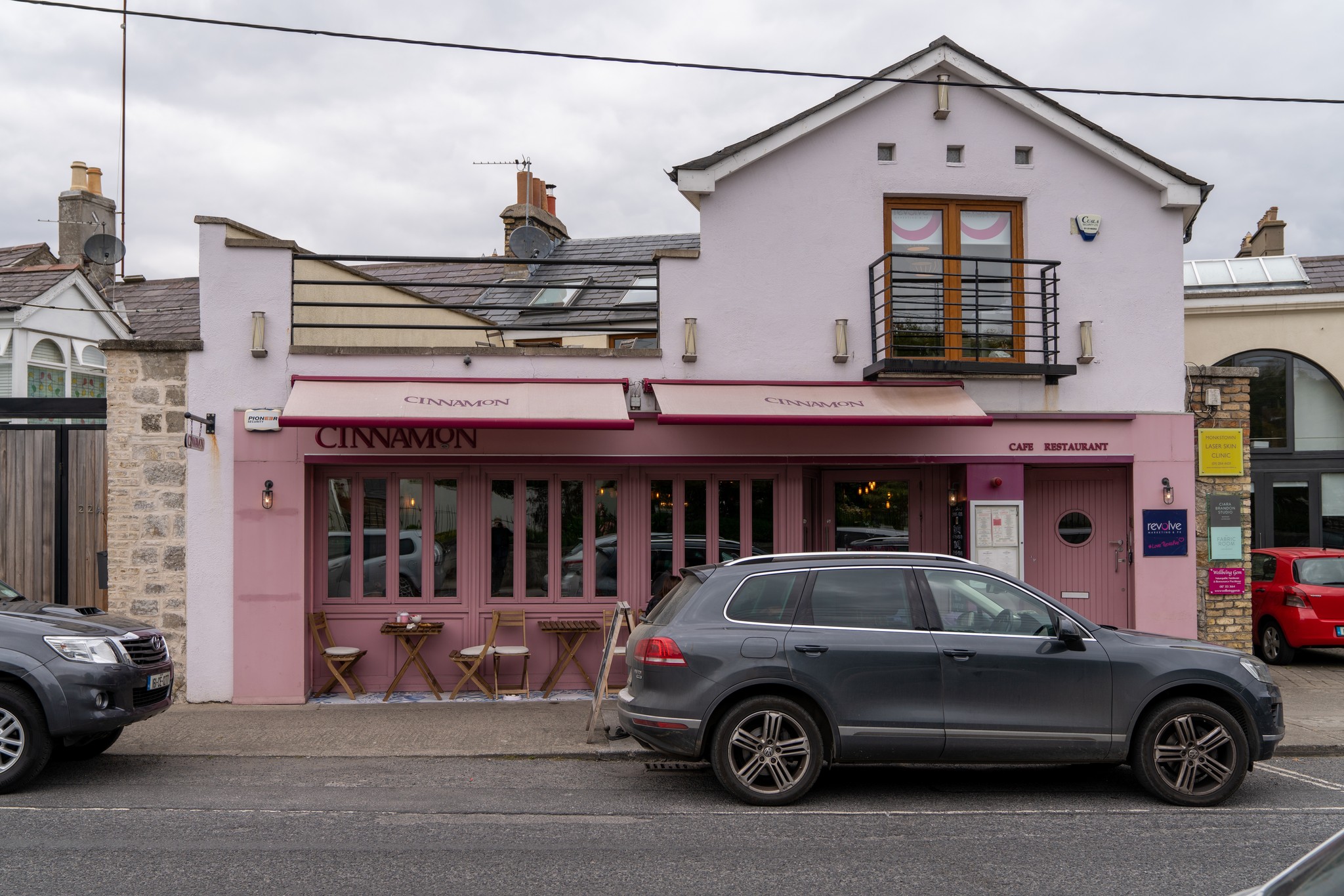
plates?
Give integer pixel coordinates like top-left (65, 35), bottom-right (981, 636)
top-left (417, 622), bottom-right (445, 627)
top-left (387, 622), bottom-right (412, 628)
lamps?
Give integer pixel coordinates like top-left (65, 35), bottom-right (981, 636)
top-left (948, 482), bottom-right (959, 506)
top-left (832, 319), bottom-right (850, 363)
top-left (1077, 320), bottom-right (1094, 364)
top-left (682, 318), bottom-right (698, 362)
top-left (1162, 478), bottom-right (1173, 505)
top-left (262, 480), bottom-right (274, 509)
top-left (904, 244), bottom-right (940, 276)
top-left (249, 311), bottom-right (268, 358)
top-left (933, 73), bottom-right (950, 120)
top-left (463, 355), bottom-right (472, 366)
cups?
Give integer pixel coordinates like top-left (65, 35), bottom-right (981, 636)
top-left (409, 616), bottom-right (422, 623)
top-left (400, 613), bottom-right (409, 623)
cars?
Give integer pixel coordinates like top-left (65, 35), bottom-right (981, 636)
top-left (542, 524), bottom-right (918, 597)
top-left (1250, 546), bottom-right (1342, 666)
top-left (325, 523), bottom-right (446, 598)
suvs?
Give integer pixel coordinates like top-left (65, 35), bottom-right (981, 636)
top-left (614, 548), bottom-right (1289, 809)
top-left (0, 579), bottom-right (176, 796)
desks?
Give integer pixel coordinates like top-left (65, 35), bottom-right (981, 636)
top-left (513, 342), bottom-right (564, 348)
top-left (380, 622), bottom-right (445, 700)
top-left (537, 620), bottom-right (601, 698)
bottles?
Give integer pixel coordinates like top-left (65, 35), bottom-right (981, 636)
top-left (396, 610), bottom-right (402, 622)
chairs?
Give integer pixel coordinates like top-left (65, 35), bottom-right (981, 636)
top-left (308, 609), bottom-right (368, 700)
top-left (475, 325), bottom-right (505, 347)
top-left (492, 609), bottom-right (531, 698)
top-left (448, 610), bottom-right (501, 700)
top-left (603, 608), bottom-right (635, 699)
top-left (619, 337), bottom-right (638, 348)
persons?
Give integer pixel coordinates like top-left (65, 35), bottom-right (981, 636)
top-left (645, 575), bottom-right (682, 617)
top-left (491, 518), bottom-right (513, 597)
